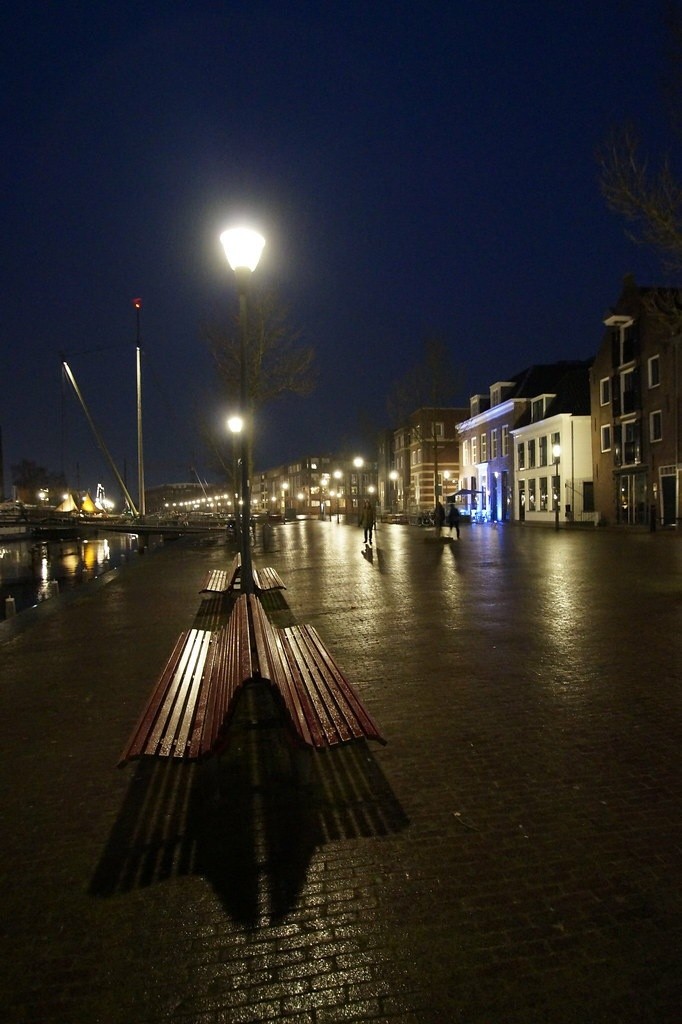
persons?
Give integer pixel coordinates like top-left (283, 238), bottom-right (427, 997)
top-left (447, 504), bottom-right (460, 540)
top-left (433, 502), bottom-right (445, 537)
top-left (360, 502), bottom-right (376, 545)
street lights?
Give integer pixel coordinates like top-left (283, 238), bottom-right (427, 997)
top-left (333, 469), bottom-right (343, 524)
top-left (227, 416), bottom-right (243, 552)
top-left (282, 483), bottom-right (290, 524)
top-left (352, 456), bottom-right (365, 527)
top-left (216, 224), bottom-right (267, 591)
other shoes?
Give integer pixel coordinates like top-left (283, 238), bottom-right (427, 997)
top-left (364, 540), bottom-right (367, 543)
top-left (369, 539), bottom-right (372, 544)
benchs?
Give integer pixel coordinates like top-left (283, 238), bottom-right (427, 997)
top-left (250, 594), bottom-right (385, 751)
top-left (118, 594), bottom-right (253, 776)
top-left (244, 552), bottom-right (287, 591)
top-left (199, 552), bottom-right (242, 593)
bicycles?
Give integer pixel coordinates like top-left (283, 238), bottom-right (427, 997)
top-left (415, 510), bottom-right (436, 528)
